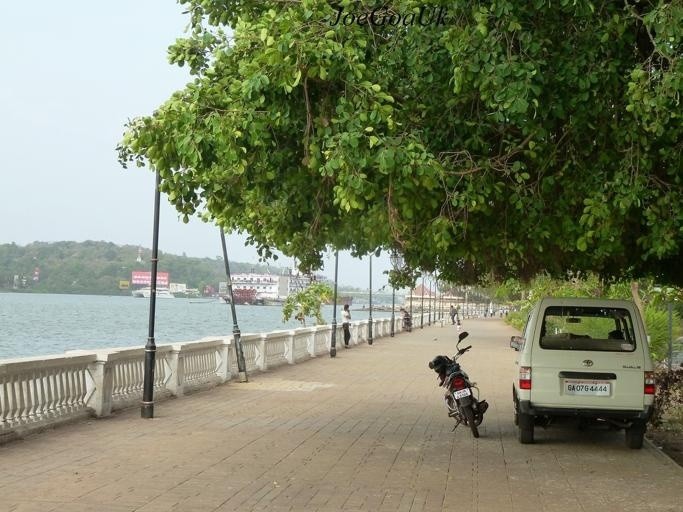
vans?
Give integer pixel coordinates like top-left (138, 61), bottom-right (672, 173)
top-left (510, 297), bottom-right (656, 449)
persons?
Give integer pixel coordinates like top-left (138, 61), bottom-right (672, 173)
top-left (400, 308), bottom-right (411, 332)
top-left (449, 305), bottom-right (456, 325)
top-left (340, 303), bottom-right (352, 349)
top-left (484, 306), bottom-right (513, 319)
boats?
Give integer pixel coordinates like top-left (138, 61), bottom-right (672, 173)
top-left (131, 286), bottom-right (175, 299)
top-left (219, 288), bottom-right (264, 305)
top-left (190, 298), bottom-right (216, 303)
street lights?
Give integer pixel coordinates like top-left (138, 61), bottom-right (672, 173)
top-left (421, 272), bottom-right (447, 328)
top-left (390, 252), bottom-right (399, 337)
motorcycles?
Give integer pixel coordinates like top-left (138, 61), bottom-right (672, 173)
top-left (403, 311), bottom-right (413, 332)
top-left (429, 332), bottom-right (488, 438)
